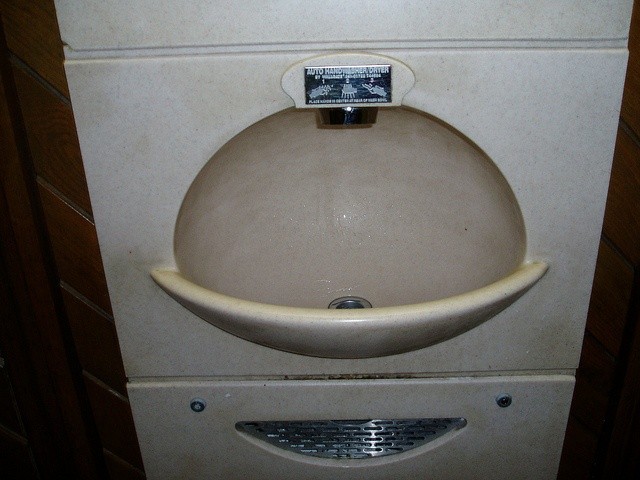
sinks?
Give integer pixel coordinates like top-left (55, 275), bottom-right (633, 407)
top-left (150, 104), bottom-right (550, 360)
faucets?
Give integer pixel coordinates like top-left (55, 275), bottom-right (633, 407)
top-left (315, 106), bottom-right (379, 128)
